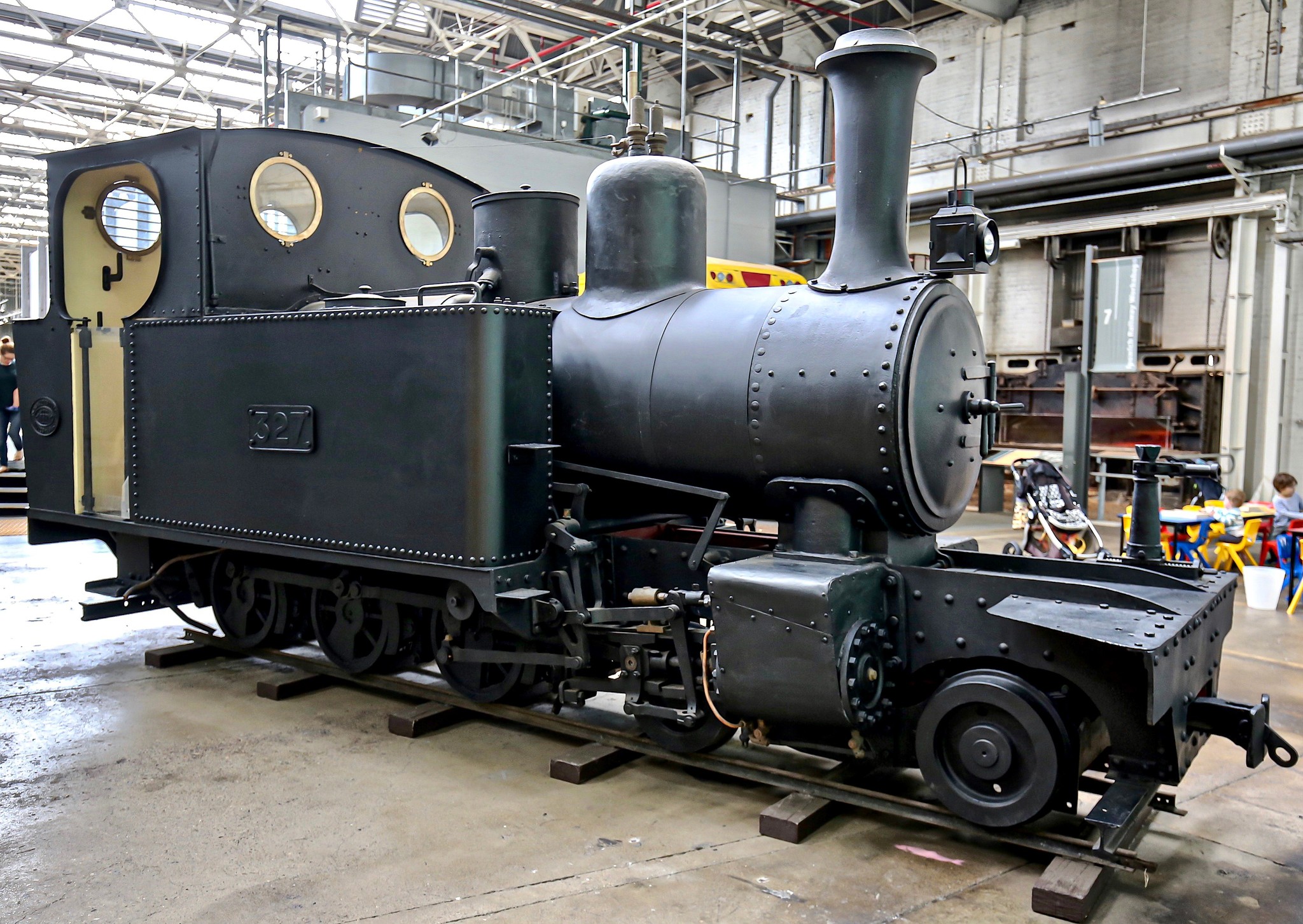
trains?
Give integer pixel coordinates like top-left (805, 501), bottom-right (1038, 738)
top-left (11, 27), bottom-right (1299, 924)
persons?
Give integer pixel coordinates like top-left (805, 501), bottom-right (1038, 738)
top-left (1271, 472), bottom-right (1303, 540)
top-left (1200, 488), bottom-right (1245, 547)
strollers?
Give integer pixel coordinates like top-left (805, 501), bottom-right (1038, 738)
top-left (1007, 457), bottom-right (1113, 563)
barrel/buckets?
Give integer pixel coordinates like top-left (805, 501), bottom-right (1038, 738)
top-left (1243, 565), bottom-right (1286, 611)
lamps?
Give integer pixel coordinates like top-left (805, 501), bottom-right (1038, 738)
top-left (421, 120), bottom-right (445, 146)
